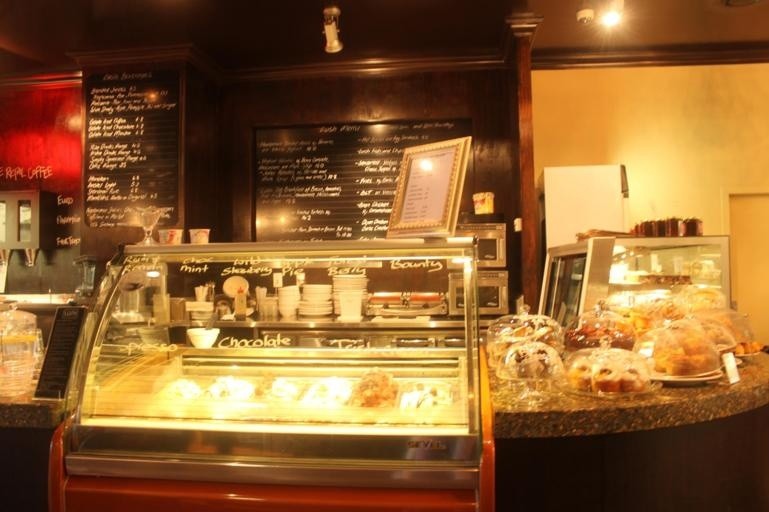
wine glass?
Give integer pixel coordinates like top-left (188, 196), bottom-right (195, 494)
top-left (123, 207), bottom-right (174, 244)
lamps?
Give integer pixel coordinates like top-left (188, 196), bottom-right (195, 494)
top-left (323, 5), bottom-right (343, 55)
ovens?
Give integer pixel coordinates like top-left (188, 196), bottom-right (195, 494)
top-left (449, 222), bottom-right (509, 316)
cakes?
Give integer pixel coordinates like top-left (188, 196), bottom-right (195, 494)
top-left (493, 316), bottom-right (708, 394)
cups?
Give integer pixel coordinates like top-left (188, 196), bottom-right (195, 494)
top-left (158, 228), bottom-right (211, 245)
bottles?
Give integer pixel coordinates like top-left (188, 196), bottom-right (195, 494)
top-left (234, 286), bottom-right (246, 321)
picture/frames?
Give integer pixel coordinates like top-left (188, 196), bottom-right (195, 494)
top-left (384, 134), bottom-right (475, 236)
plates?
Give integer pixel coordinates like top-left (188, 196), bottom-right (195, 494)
top-left (298, 275), bottom-right (369, 315)
top-left (651, 327), bottom-right (761, 387)
top-left (222, 276), bottom-right (250, 297)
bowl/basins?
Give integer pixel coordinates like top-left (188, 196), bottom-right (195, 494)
top-left (279, 286), bottom-right (300, 316)
top-left (187, 328), bottom-right (220, 348)
top-left (137, 327), bottom-right (169, 344)
